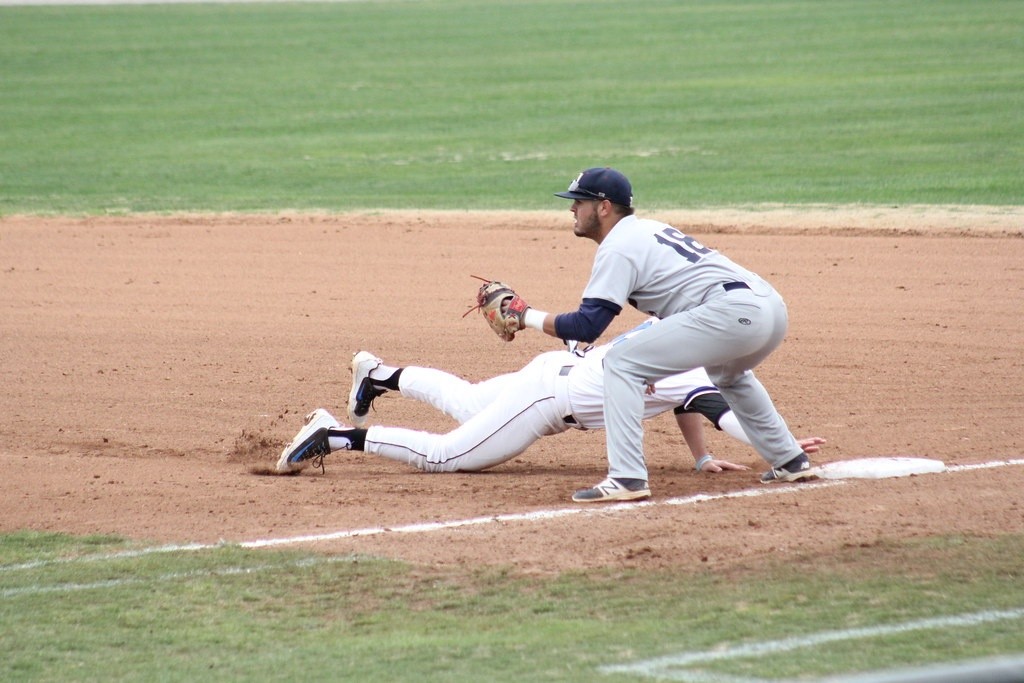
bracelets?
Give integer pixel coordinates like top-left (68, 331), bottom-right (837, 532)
top-left (695, 455), bottom-right (711, 473)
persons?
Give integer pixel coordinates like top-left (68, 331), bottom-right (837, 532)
top-left (275, 316), bottom-right (826, 474)
top-left (476, 167), bottom-right (818, 503)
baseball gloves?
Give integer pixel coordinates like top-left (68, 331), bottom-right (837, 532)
top-left (476, 279), bottom-right (529, 344)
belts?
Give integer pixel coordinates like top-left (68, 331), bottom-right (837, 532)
top-left (723, 283), bottom-right (749, 292)
top-left (556, 365), bottom-right (589, 431)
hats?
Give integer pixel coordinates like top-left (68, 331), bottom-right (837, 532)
top-left (553, 166), bottom-right (633, 206)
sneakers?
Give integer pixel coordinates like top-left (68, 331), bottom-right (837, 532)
top-left (761, 452), bottom-right (820, 484)
top-left (572, 477), bottom-right (651, 502)
top-left (276, 408), bottom-right (338, 474)
top-left (348, 351), bottom-right (388, 423)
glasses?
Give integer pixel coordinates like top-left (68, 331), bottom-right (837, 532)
top-left (568, 179), bottom-right (611, 200)
